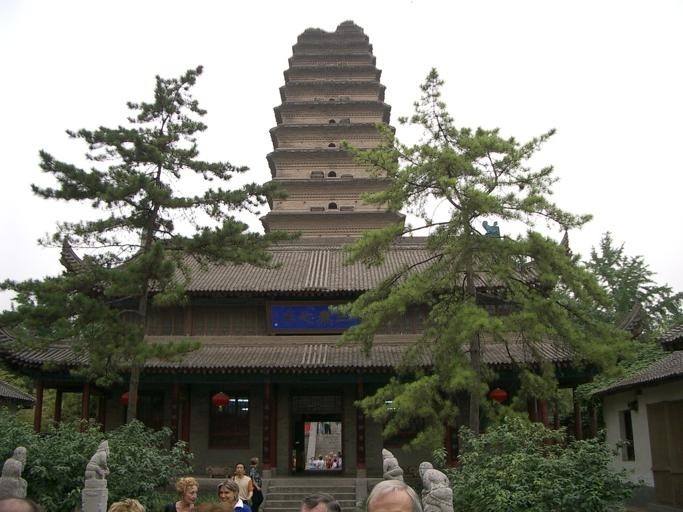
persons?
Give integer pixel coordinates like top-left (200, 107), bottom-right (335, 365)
top-left (107, 496), bottom-right (146, 512)
top-left (232, 462), bottom-right (252, 505)
top-left (300, 493), bottom-right (341, 512)
top-left (0, 495), bottom-right (43, 512)
top-left (249, 455), bottom-right (264, 512)
top-left (309, 451), bottom-right (342, 470)
top-left (217, 479), bottom-right (253, 512)
top-left (369, 479), bottom-right (423, 511)
top-left (164, 476), bottom-right (200, 511)
top-left (323, 422), bottom-right (332, 434)
top-left (196, 501), bottom-right (236, 512)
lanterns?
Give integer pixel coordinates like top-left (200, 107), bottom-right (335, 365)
top-left (121, 391), bottom-right (128, 404)
top-left (489, 387), bottom-right (507, 404)
top-left (212, 391), bottom-right (229, 411)
